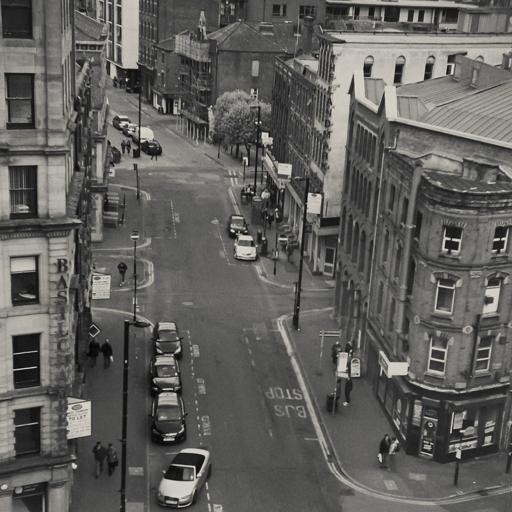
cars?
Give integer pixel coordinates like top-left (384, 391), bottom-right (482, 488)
top-left (227, 213), bottom-right (258, 261)
top-left (127, 85), bottom-right (141, 93)
top-left (112, 115), bottom-right (162, 155)
top-left (150, 321), bottom-right (212, 509)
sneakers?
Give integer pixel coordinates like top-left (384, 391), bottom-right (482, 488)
top-left (343, 401), bottom-right (351, 407)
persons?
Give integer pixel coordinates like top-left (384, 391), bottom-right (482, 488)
top-left (118, 262), bottom-right (127, 286)
top-left (343, 374), bottom-right (353, 407)
top-left (151, 144), bottom-right (160, 160)
top-left (241, 184), bottom-right (283, 230)
top-left (121, 141), bottom-right (126, 153)
top-left (87, 338), bottom-right (101, 367)
top-left (332, 340), bottom-right (354, 369)
top-left (106, 444), bottom-right (117, 476)
top-left (92, 441), bottom-right (107, 479)
top-left (101, 339), bottom-right (113, 369)
top-left (112, 76), bottom-right (126, 89)
top-left (126, 140), bottom-right (130, 153)
top-left (379, 433), bottom-right (391, 469)
top-left (388, 436), bottom-right (400, 472)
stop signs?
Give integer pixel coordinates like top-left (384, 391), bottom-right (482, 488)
top-left (278, 234), bottom-right (288, 246)
top-left (129, 231), bottom-right (140, 241)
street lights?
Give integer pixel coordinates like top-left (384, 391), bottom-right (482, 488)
top-left (120, 318), bottom-right (151, 512)
top-left (251, 105), bottom-right (261, 195)
top-left (293, 176), bottom-right (309, 328)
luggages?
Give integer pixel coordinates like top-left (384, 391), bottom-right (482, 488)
top-left (327, 387), bottom-right (336, 412)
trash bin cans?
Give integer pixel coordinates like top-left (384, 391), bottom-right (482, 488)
top-left (158, 107), bottom-right (163, 114)
top-left (133, 149), bottom-right (140, 157)
top-left (242, 195), bottom-right (248, 204)
top-left (326, 393), bottom-right (340, 412)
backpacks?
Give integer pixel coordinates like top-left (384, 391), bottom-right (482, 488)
top-left (395, 443), bottom-right (401, 453)
